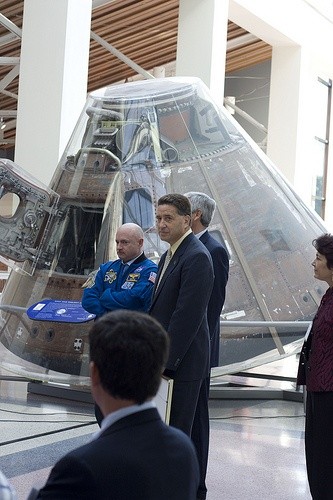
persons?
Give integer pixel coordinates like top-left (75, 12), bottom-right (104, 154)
top-left (296, 233), bottom-right (333, 500)
top-left (81, 223), bottom-right (158, 429)
top-left (149, 194), bottom-right (215, 439)
top-left (183, 191), bottom-right (229, 500)
top-left (34, 309), bottom-right (199, 500)
